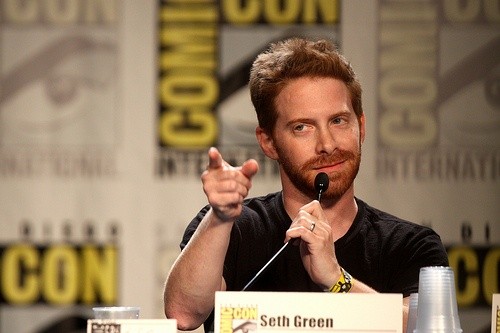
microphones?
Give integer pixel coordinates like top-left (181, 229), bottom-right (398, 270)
top-left (241, 172), bottom-right (330, 292)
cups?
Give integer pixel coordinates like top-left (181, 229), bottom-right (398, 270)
top-left (406, 266), bottom-right (464, 333)
top-left (92, 307), bottom-right (141, 319)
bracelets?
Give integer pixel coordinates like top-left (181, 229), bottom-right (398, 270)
top-left (324, 267), bottom-right (353, 294)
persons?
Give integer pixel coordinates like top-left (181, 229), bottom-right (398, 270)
top-left (164, 38), bottom-right (450, 333)
